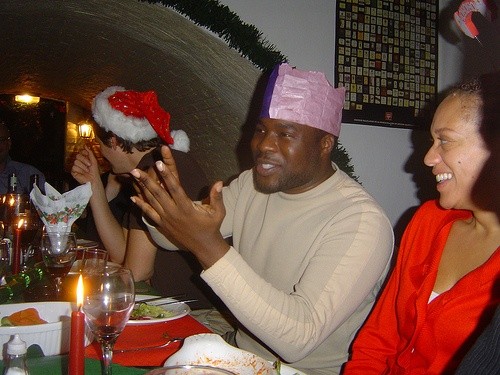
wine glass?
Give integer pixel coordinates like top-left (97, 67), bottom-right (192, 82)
top-left (13, 213), bottom-right (38, 269)
top-left (80, 265), bottom-right (135, 375)
top-left (41, 233), bottom-right (77, 301)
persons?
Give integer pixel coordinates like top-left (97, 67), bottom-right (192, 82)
top-left (342, 73), bottom-right (500, 375)
top-left (130, 67), bottom-right (396, 375)
top-left (70, 86), bottom-right (221, 309)
top-left (0, 123), bottom-right (46, 219)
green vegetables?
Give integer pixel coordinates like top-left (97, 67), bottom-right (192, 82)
top-left (131, 303), bottom-right (174, 318)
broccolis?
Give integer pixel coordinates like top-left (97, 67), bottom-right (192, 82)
top-left (1, 316), bottom-right (14, 327)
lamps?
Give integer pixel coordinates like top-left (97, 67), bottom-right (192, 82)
top-left (14, 94), bottom-right (40, 103)
top-left (78, 120), bottom-right (92, 138)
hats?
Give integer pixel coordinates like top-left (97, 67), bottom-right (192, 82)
top-left (258, 62), bottom-right (347, 137)
top-left (91, 87), bottom-right (192, 154)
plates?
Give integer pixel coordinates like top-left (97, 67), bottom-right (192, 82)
top-left (68, 259), bottom-right (122, 274)
top-left (70, 239), bottom-right (98, 250)
top-left (126, 295), bottom-right (190, 326)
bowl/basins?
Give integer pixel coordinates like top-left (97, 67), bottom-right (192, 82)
top-left (269, 361), bottom-right (307, 375)
top-left (0, 301), bottom-right (94, 361)
top-left (163, 333), bottom-right (277, 375)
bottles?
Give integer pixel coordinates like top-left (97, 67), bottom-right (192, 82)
top-left (4, 172), bottom-right (20, 240)
top-left (30, 174), bottom-right (39, 191)
top-left (5, 333), bottom-right (30, 375)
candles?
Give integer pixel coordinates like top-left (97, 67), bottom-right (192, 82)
top-left (12, 219), bottom-right (24, 276)
top-left (68, 274), bottom-right (84, 374)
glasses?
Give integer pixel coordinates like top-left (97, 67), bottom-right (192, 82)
top-left (0, 137), bottom-right (11, 142)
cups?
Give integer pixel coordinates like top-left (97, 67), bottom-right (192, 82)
top-left (42, 225), bottom-right (72, 233)
top-left (144, 365), bottom-right (236, 375)
top-left (82, 249), bottom-right (109, 268)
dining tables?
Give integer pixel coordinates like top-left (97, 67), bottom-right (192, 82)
top-left (0, 278), bottom-right (213, 375)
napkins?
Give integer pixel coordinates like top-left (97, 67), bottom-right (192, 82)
top-left (30, 181), bottom-right (93, 255)
